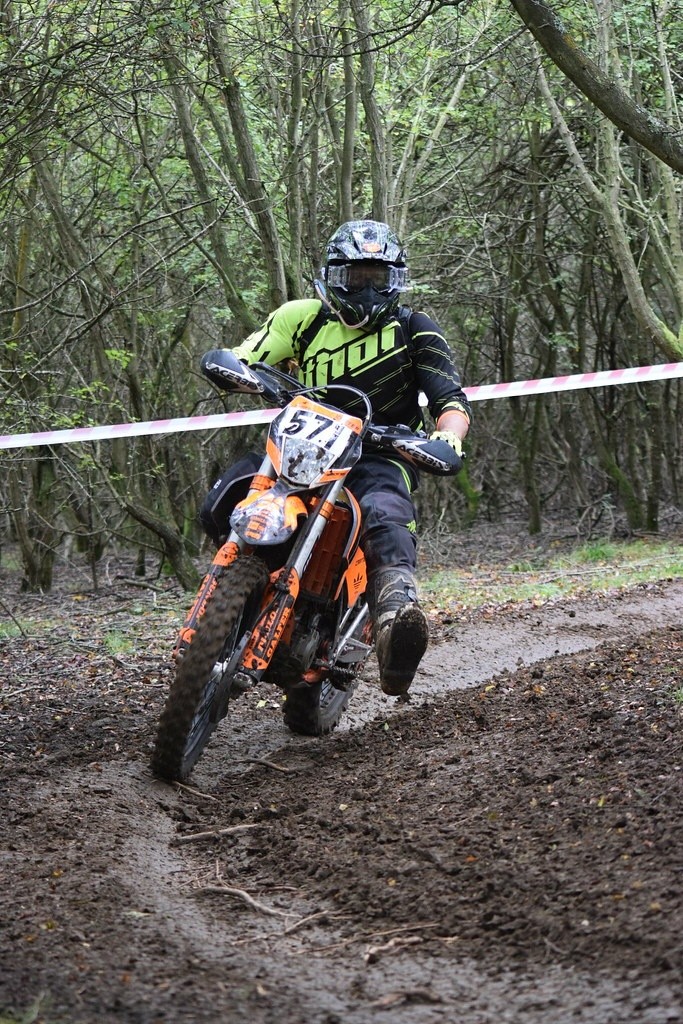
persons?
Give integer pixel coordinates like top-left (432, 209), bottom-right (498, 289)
top-left (200, 220), bottom-right (474, 696)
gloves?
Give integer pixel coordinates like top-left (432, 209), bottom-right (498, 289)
top-left (430, 430), bottom-right (462, 457)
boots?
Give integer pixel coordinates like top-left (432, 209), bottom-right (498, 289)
top-left (368, 566), bottom-right (429, 696)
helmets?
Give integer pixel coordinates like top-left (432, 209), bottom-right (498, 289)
top-left (314, 220), bottom-right (408, 332)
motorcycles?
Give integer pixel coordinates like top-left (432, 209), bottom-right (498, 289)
top-left (150, 351), bottom-right (467, 784)
